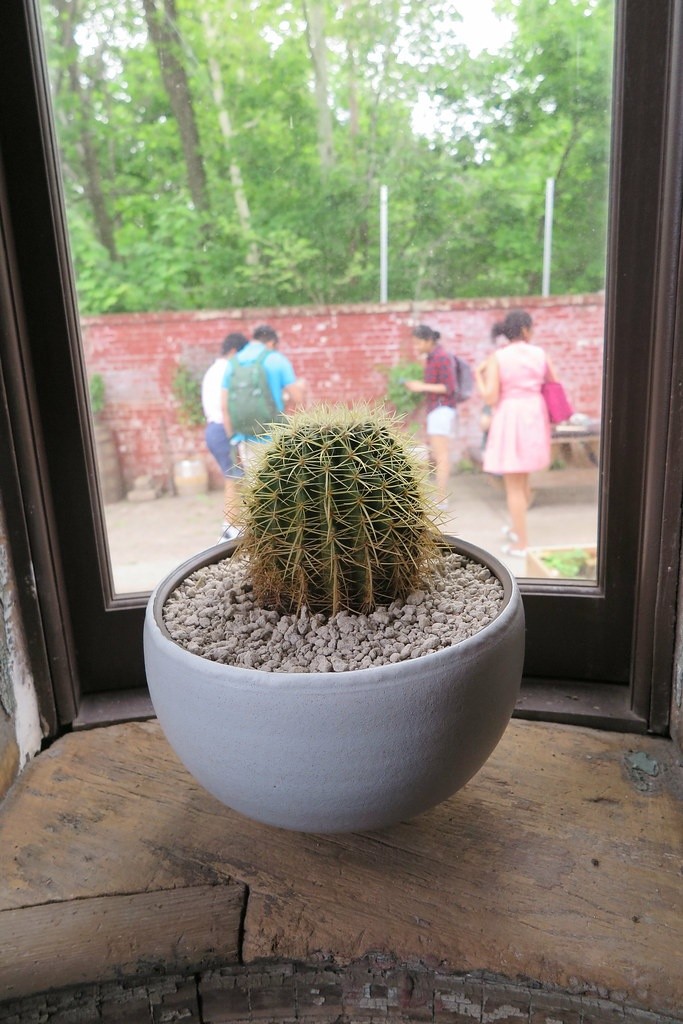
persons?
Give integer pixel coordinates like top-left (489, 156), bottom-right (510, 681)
top-left (403, 325), bottom-right (458, 512)
top-left (201, 326), bottom-right (306, 544)
top-left (475, 309), bottom-right (557, 560)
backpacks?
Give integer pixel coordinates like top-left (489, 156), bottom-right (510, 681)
top-left (225, 349), bottom-right (278, 437)
top-left (437, 353), bottom-right (472, 402)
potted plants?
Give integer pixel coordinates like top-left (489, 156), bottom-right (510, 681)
top-left (169, 368), bottom-right (210, 496)
top-left (87, 372), bottom-right (123, 506)
top-left (385, 362), bottom-right (431, 481)
top-left (145, 396), bottom-right (528, 834)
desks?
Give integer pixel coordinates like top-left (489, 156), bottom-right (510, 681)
top-left (550, 426), bottom-right (600, 496)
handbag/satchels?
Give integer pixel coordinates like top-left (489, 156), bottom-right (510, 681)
top-left (542, 357), bottom-right (573, 425)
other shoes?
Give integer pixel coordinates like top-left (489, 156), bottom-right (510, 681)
top-left (501, 529), bottom-right (531, 557)
